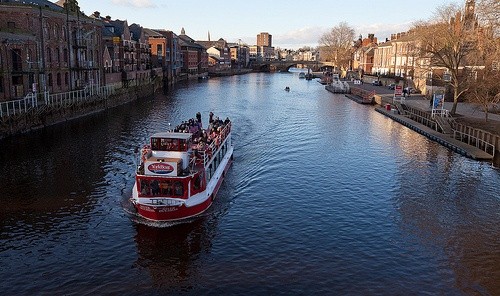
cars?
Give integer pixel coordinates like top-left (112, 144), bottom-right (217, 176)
top-left (372, 80), bottom-right (381, 85)
top-left (388, 84), bottom-right (395, 89)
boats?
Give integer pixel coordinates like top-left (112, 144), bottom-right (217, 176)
top-left (299, 72), bottom-right (313, 78)
top-left (198, 76), bottom-right (210, 79)
top-left (130, 112), bottom-right (233, 222)
top-left (285, 87), bottom-right (290, 90)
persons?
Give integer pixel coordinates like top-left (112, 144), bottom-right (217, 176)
top-left (404, 89), bottom-right (407, 97)
top-left (430, 95), bottom-right (434, 109)
top-left (408, 89), bottom-right (410, 96)
top-left (434, 99), bottom-right (439, 109)
top-left (140, 110), bottom-right (231, 170)
